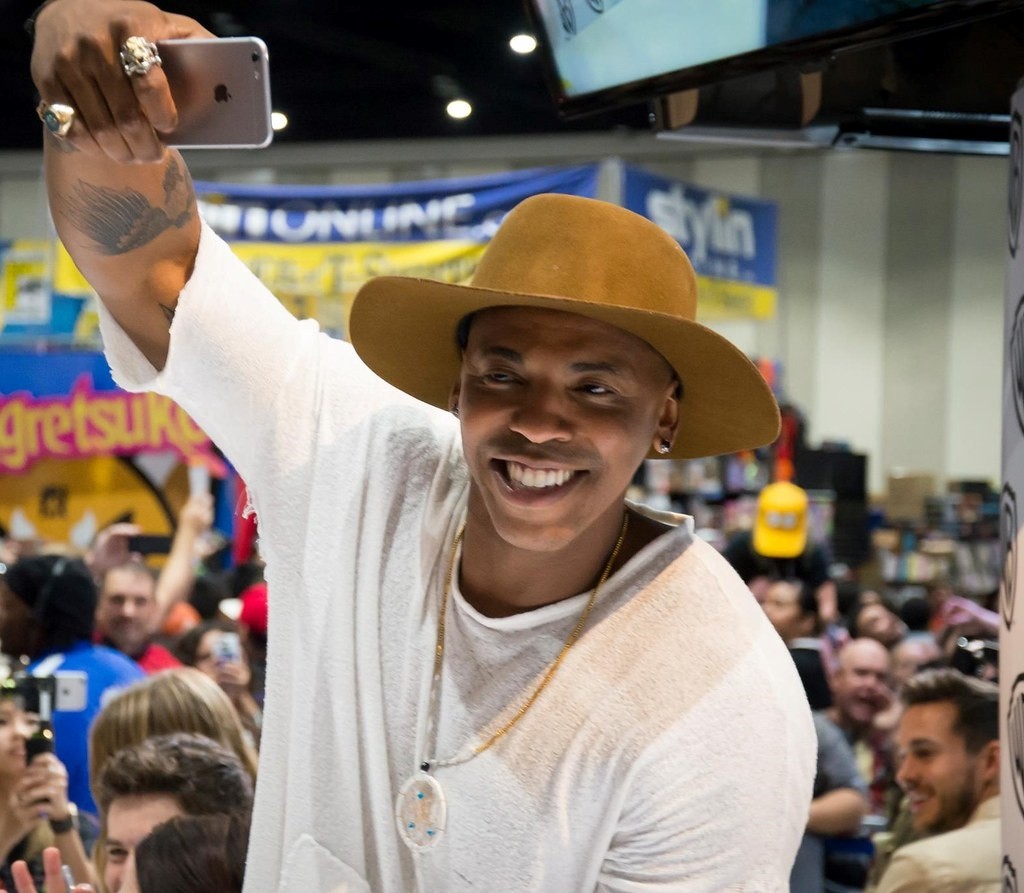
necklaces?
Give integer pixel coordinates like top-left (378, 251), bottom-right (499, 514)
top-left (395, 501), bottom-right (630, 855)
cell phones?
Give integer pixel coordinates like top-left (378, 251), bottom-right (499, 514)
top-left (212, 633), bottom-right (240, 662)
top-left (128, 536), bottom-right (173, 555)
top-left (26, 733), bottom-right (54, 768)
top-left (154, 37), bottom-right (273, 149)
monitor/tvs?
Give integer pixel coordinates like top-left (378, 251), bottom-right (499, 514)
top-left (525, 0), bottom-right (1024, 117)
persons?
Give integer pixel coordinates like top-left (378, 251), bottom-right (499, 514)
top-left (31, 0), bottom-right (816, 893)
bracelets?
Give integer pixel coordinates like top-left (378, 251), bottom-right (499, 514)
top-left (46, 800), bottom-right (79, 832)
top-left (0, 482), bottom-right (1001, 893)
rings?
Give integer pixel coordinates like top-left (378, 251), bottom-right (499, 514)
top-left (121, 34), bottom-right (164, 76)
top-left (34, 99), bottom-right (75, 140)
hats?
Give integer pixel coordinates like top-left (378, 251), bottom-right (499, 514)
top-left (8, 556), bottom-right (97, 642)
top-left (753, 481), bottom-right (811, 559)
top-left (346, 190), bottom-right (783, 463)
